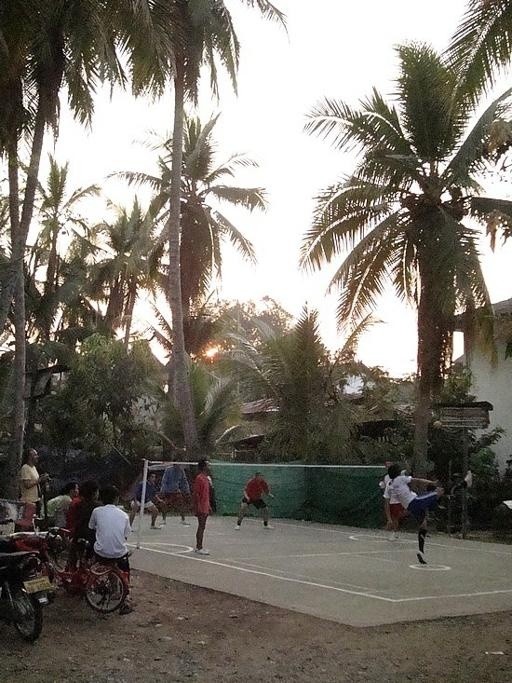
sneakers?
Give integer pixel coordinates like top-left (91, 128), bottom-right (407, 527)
top-left (417, 551), bottom-right (427, 564)
top-left (263, 524), bottom-right (274, 529)
top-left (181, 520), bottom-right (192, 525)
top-left (150, 520), bottom-right (166, 529)
top-left (234, 524), bottom-right (240, 530)
top-left (388, 532), bottom-right (399, 542)
top-left (195, 546), bottom-right (209, 555)
top-left (464, 471), bottom-right (473, 488)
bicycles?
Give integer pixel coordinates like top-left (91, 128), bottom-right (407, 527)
top-left (31, 513), bottom-right (129, 614)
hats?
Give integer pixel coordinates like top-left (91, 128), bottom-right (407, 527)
top-left (255, 471), bottom-right (264, 476)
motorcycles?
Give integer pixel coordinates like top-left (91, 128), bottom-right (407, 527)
top-left (0, 541), bottom-right (53, 643)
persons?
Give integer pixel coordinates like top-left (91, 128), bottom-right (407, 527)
top-left (234, 471), bottom-right (275, 530)
top-left (159, 457), bottom-right (191, 526)
top-left (379, 458), bottom-right (413, 542)
top-left (128, 473), bottom-right (162, 532)
top-left (382, 464), bottom-right (473, 564)
top-left (192, 460), bottom-right (213, 555)
top-left (18, 447), bottom-right (135, 616)
